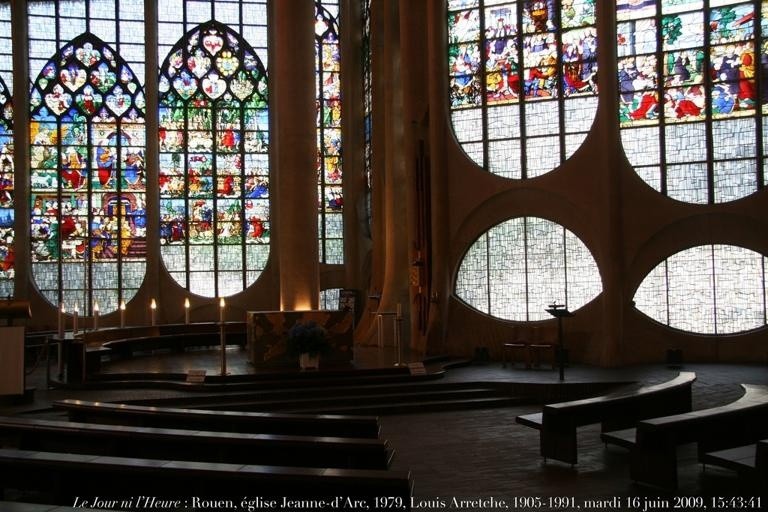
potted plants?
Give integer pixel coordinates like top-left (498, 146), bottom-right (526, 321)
top-left (288, 318), bottom-right (333, 371)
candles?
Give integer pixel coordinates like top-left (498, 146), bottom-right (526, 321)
top-left (60, 312), bottom-right (65, 337)
top-left (186, 307), bottom-right (190, 324)
top-left (74, 310), bottom-right (78, 333)
top-left (120, 310), bottom-right (124, 329)
top-left (151, 309), bottom-right (155, 326)
top-left (219, 306), bottom-right (225, 322)
top-left (94, 310), bottom-right (97, 329)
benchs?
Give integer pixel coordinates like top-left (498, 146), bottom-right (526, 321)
top-left (47, 319), bottom-right (245, 386)
top-left (515, 366), bottom-right (768, 504)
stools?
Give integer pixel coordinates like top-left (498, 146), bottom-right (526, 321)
top-left (529, 343), bottom-right (552, 371)
top-left (502, 343), bottom-right (526, 370)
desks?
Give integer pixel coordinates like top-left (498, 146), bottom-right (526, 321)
top-left (246, 309), bottom-right (356, 371)
